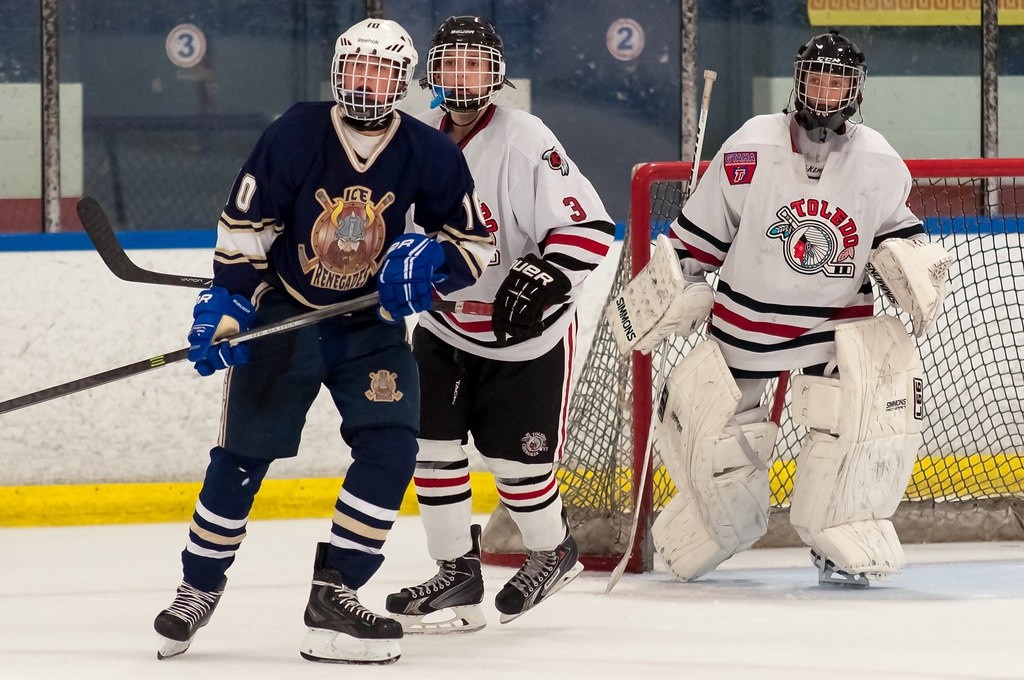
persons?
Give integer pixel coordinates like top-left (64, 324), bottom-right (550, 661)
top-left (385, 14), bottom-right (614, 635)
top-left (607, 30), bottom-right (949, 587)
top-left (153, 16), bottom-right (496, 667)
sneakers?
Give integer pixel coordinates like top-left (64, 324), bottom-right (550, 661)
top-left (495, 508), bottom-right (584, 624)
top-left (154, 575), bottom-right (227, 660)
top-left (299, 543), bottom-right (403, 665)
top-left (386, 526), bottom-right (486, 635)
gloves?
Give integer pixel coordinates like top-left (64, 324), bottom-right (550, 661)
top-left (379, 233), bottom-right (449, 320)
top-left (187, 287), bottom-right (254, 376)
top-left (492, 253), bottom-right (572, 343)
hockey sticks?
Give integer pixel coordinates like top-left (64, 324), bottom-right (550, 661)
top-left (606, 69), bottom-right (718, 595)
top-left (0, 290), bottom-right (380, 414)
top-left (76, 196), bottom-right (493, 317)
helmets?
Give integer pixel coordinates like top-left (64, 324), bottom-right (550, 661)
top-left (427, 16), bottom-right (505, 113)
top-left (331, 18), bottom-right (418, 121)
top-left (795, 31), bottom-right (868, 131)
